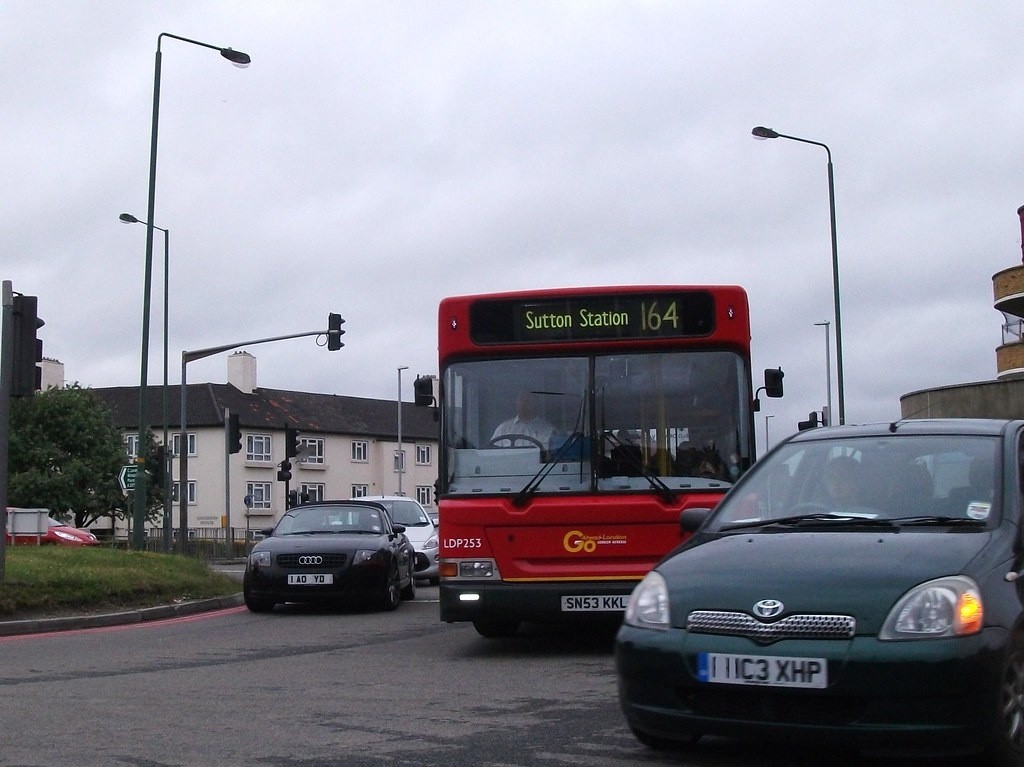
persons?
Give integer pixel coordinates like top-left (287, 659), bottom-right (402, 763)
top-left (490, 387), bottom-right (563, 449)
top-left (813, 456), bottom-right (892, 519)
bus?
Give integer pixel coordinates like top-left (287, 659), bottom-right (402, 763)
top-left (413, 280), bottom-right (784, 640)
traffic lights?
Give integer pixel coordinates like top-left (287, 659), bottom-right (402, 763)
top-left (798, 411), bottom-right (818, 431)
top-left (301, 492), bottom-right (310, 504)
top-left (328, 312), bottom-right (346, 351)
top-left (228, 412), bottom-right (243, 454)
top-left (289, 489), bottom-right (297, 509)
top-left (286, 429), bottom-right (301, 456)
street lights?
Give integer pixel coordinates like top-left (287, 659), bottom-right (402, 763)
top-left (133, 29), bottom-right (253, 553)
top-left (119, 213), bottom-right (174, 552)
top-left (396, 365), bottom-right (410, 497)
top-left (765, 415), bottom-right (775, 453)
top-left (814, 319), bottom-right (831, 428)
top-left (752, 125), bottom-right (844, 425)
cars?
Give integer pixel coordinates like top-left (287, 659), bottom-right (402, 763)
top-left (5, 515), bottom-right (102, 549)
top-left (244, 497), bottom-right (415, 614)
top-left (329, 494), bottom-right (440, 586)
top-left (616, 416), bottom-right (1024, 767)
